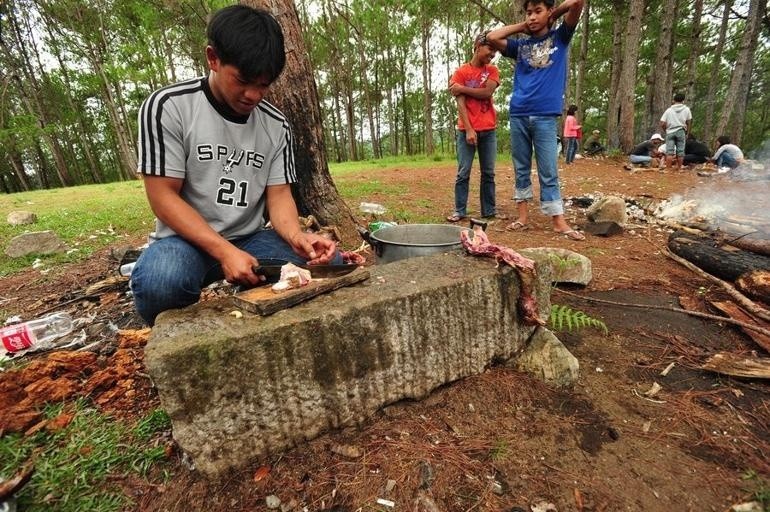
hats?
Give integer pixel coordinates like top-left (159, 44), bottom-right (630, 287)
top-left (650, 133), bottom-right (665, 141)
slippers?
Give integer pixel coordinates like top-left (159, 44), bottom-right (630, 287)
top-left (561, 230), bottom-right (586, 241)
top-left (505, 222), bottom-right (531, 232)
top-left (446, 215), bottom-right (463, 223)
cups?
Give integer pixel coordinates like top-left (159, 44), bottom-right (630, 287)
top-left (118, 261), bottom-right (137, 278)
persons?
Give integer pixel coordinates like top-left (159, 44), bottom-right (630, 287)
top-left (447, 31), bottom-right (499, 222)
top-left (564, 105), bottom-right (583, 164)
top-left (130, 4), bottom-right (343, 328)
top-left (486, 0), bottom-right (586, 241)
top-left (630, 133), bottom-right (665, 164)
top-left (711, 136), bottom-right (744, 169)
top-left (660, 94), bottom-right (692, 169)
top-left (583, 130), bottom-right (601, 153)
top-left (683, 134), bottom-right (711, 166)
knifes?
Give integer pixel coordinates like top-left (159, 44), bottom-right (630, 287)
top-left (245, 263), bottom-right (360, 282)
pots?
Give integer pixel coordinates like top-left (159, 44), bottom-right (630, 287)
top-left (357, 223), bottom-right (482, 265)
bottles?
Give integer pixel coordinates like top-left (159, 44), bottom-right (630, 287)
top-left (0, 311), bottom-right (76, 351)
top-left (366, 220), bottom-right (393, 231)
top-left (359, 202), bottom-right (391, 216)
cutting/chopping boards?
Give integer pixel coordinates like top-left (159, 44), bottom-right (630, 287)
top-left (232, 267), bottom-right (369, 315)
